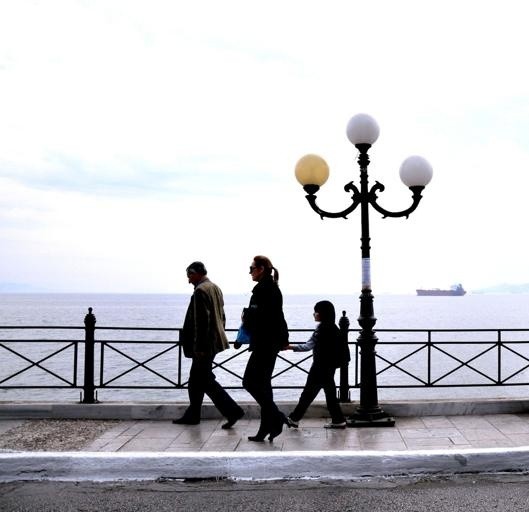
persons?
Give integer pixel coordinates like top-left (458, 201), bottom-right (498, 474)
top-left (172, 261), bottom-right (244, 429)
top-left (234, 255), bottom-right (289, 442)
top-left (281, 300), bottom-right (347, 430)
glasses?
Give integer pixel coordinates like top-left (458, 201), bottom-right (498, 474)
top-left (249, 266), bottom-right (259, 274)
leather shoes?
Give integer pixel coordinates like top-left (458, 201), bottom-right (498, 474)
top-left (221, 407), bottom-right (245, 429)
top-left (172, 412), bottom-right (201, 425)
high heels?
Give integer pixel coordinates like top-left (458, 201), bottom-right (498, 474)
top-left (268, 410), bottom-right (291, 441)
top-left (248, 425), bottom-right (273, 441)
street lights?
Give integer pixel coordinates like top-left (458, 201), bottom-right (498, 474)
top-left (296, 112), bottom-right (433, 427)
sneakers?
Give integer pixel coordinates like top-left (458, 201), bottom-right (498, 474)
top-left (324, 419), bottom-right (346, 429)
top-left (286, 414), bottom-right (298, 428)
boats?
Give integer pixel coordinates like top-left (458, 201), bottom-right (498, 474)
top-left (416, 284), bottom-right (466, 296)
